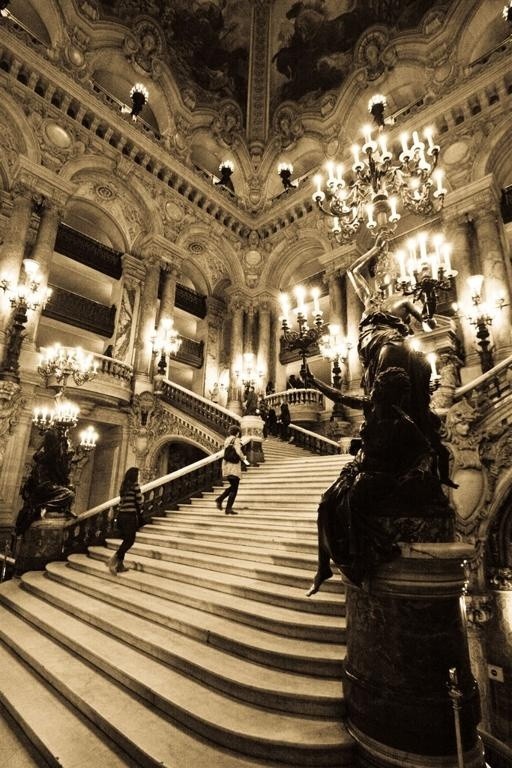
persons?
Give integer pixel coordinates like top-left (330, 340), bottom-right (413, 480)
top-left (107, 465), bottom-right (145, 575)
top-left (216, 425), bottom-right (249, 514)
top-left (246, 383), bottom-right (294, 442)
top-left (287, 374), bottom-right (310, 402)
top-left (103, 344), bottom-right (113, 368)
top-left (266, 379), bottom-right (274, 394)
top-left (302, 366), bottom-right (441, 593)
top-left (24, 425), bottom-right (77, 517)
top-left (345, 229), bottom-right (462, 492)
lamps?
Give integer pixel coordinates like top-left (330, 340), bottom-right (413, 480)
top-left (215, 157), bottom-right (238, 173)
top-left (392, 231), bottom-right (459, 300)
top-left (276, 158), bottom-right (294, 180)
top-left (273, 281), bottom-right (326, 366)
top-left (36, 343), bottom-right (100, 393)
top-left (306, 132), bottom-right (448, 240)
top-left (76, 424), bottom-right (103, 446)
top-left (32, 399), bottom-right (79, 429)
top-left (1, 252), bottom-right (55, 316)
top-left (230, 352), bottom-right (269, 395)
top-left (126, 77), bottom-right (151, 105)
top-left (457, 272), bottom-right (508, 336)
top-left (407, 335), bottom-right (443, 393)
top-left (142, 316), bottom-right (183, 375)
top-left (209, 368), bottom-right (228, 396)
top-left (319, 323), bottom-right (358, 364)
top-left (365, 93), bottom-right (386, 113)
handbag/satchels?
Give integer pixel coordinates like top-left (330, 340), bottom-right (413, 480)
top-left (224, 445), bottom-right (240, 463)
